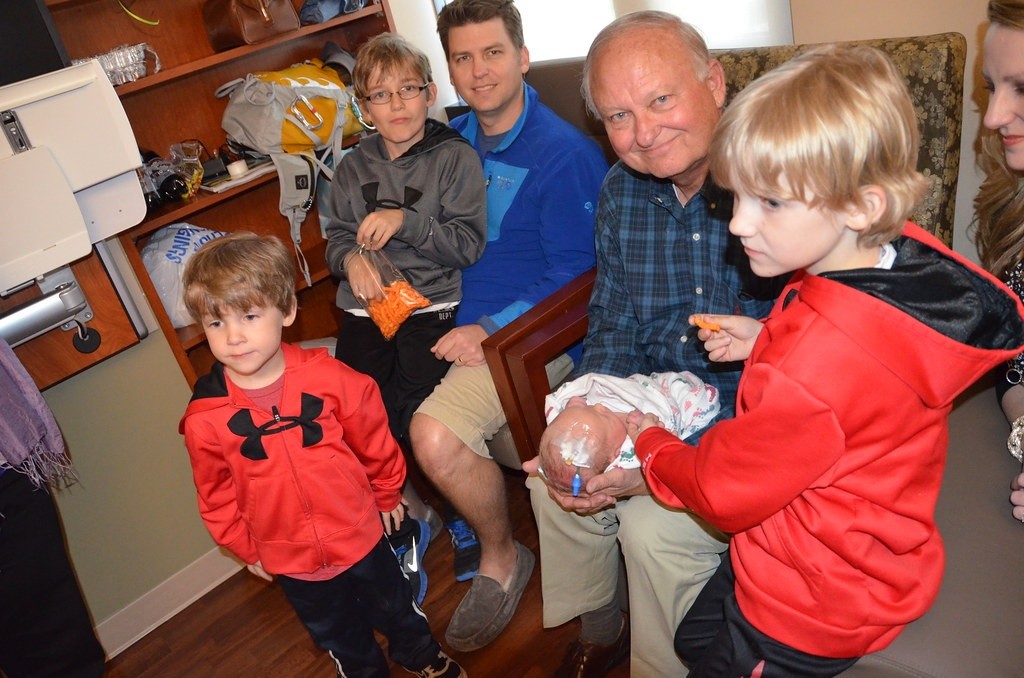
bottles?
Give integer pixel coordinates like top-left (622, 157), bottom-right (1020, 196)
top-left (135, 144), bottom-right (188, 216)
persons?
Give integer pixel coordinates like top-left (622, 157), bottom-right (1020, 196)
top-left (410, 1), bottom-right (610, 651)
top-left (527, 9), bottom-right (790, 677)
top-left (627, 40), bottom-right (1024, 678)
top-left (178, 232), bottom-right (467, 678)
top-left (0, 336), bottom-right (107, 678)
top-left (966, 0), bottom-right (1024, 529)
top-left (325, 31), bottom-right (482, 606)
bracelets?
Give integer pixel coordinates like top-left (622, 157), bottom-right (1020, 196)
top-left (1007, 416), bottom-right (1024, 464)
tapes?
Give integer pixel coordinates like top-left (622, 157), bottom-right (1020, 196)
top-left (226, 159), bottom-right (248, 176)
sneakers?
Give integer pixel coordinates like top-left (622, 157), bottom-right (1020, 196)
top-left (401, 647), bottom-right (468, 678)
top-left (443, 517), bottom-right (482, 582)
top-left (445, 540), bottom-right (536, 651)
top-left (387, 518), bottom-right (430, 605)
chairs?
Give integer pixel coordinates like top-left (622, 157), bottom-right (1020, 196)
top-left (485, 33), bottom-right (967, 556)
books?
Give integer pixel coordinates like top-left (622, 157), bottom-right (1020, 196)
top-left (198, 158), bottom-right (277, 194)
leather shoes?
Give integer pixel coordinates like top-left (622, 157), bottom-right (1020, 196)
top-left (553, 609), bottom-right (630, 678)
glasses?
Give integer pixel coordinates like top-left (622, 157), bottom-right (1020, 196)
top-left (366, 82), bottom-right (429, 105)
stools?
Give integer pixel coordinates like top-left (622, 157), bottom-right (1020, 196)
top-left (834, 377), bottom-right (1024, 678)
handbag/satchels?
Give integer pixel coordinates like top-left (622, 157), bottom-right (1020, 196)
top-left (140, 221), bottom-right (232, 329)
top-left (343, 85), bottom-right (377, 136)
top-left (215, 58), bottom-right (373, 156)
top-left (203, 0), bottom-right (300, 51)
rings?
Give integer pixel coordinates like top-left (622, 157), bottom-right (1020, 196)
top-left (458, 357), bottom-right (466, 365)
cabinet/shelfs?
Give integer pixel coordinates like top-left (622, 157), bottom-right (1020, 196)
top-left (39, 0), bottom-right (428, 393)
